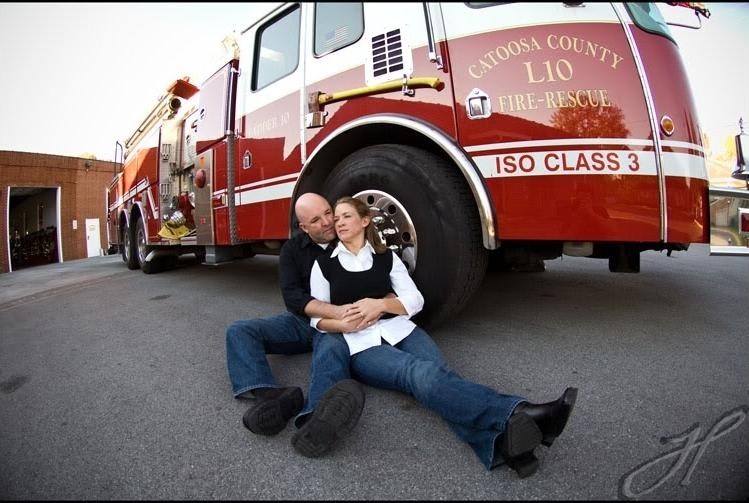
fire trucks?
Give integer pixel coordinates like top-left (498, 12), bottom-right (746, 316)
top-left (103, 2), bottom-right (749, 338)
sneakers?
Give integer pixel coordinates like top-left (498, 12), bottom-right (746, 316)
top-left (291, 378), bottom-right (365, 457)
top-left (242, 386), bottom-right (304, 436)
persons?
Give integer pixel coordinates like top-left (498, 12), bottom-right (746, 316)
top-left (309, 196), bottom-right (580, 479)
top-left (226, 191), bottom-right (397, 458)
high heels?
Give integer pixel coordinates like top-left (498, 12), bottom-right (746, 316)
top-left (513, 386), bottom-right (578, 447)
top-left (501, 412), bottom-right (544, 479)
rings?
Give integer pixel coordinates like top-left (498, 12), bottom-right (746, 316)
top-left (360, 312), bottom-right (364, 317)
